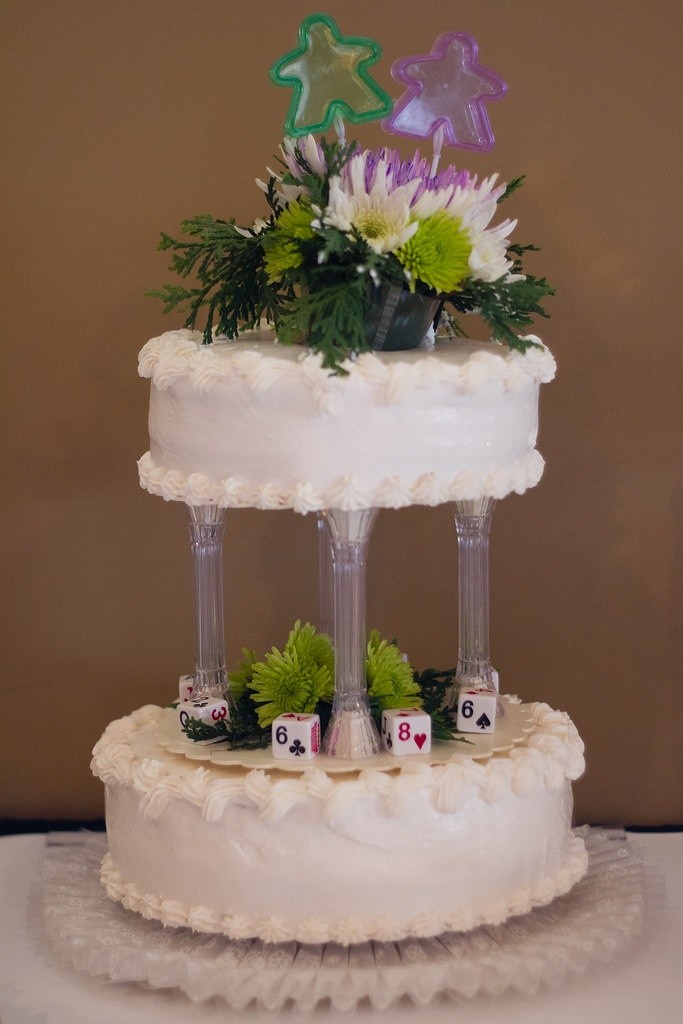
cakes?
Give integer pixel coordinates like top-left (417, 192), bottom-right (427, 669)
top-left (88, 15), bottom-right (586, 946)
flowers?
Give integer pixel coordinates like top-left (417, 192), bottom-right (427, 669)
top-left (179, 620), bottom-right (461, 754)
top-left (153, 141), bottom-right (548, 371)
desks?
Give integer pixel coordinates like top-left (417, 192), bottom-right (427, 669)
top-left (0, 832), bottom-right (683, 1024)
